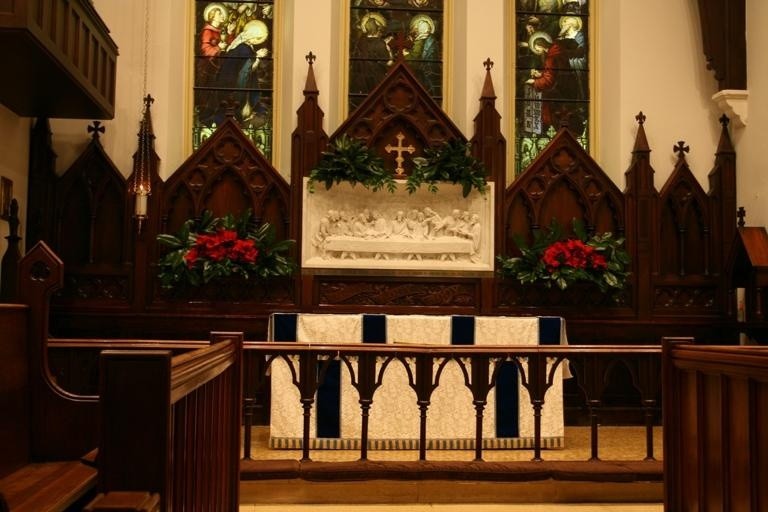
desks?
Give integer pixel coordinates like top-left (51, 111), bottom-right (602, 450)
top-left (265, 313), bottom-right (573, 450)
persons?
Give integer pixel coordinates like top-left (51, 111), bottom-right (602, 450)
top-left (514, 1), bottom-right (591, 138)
top-left (196, 2), bottom-right (272, 129)
top-left (351, 0), bottom-right (442, 95)
top-left (314, 206), bottom-right (485, 265)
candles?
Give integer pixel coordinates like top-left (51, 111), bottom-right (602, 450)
top-left (134, 183), bottom-right (149, 216)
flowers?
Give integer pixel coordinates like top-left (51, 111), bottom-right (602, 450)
top-left (144, 209), bottom-right (297, 293)
top-left (498, 216), bottom-right (633, 306)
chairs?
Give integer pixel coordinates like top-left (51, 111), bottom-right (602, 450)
top-left (0, 239), bottom-right (99, 512)
